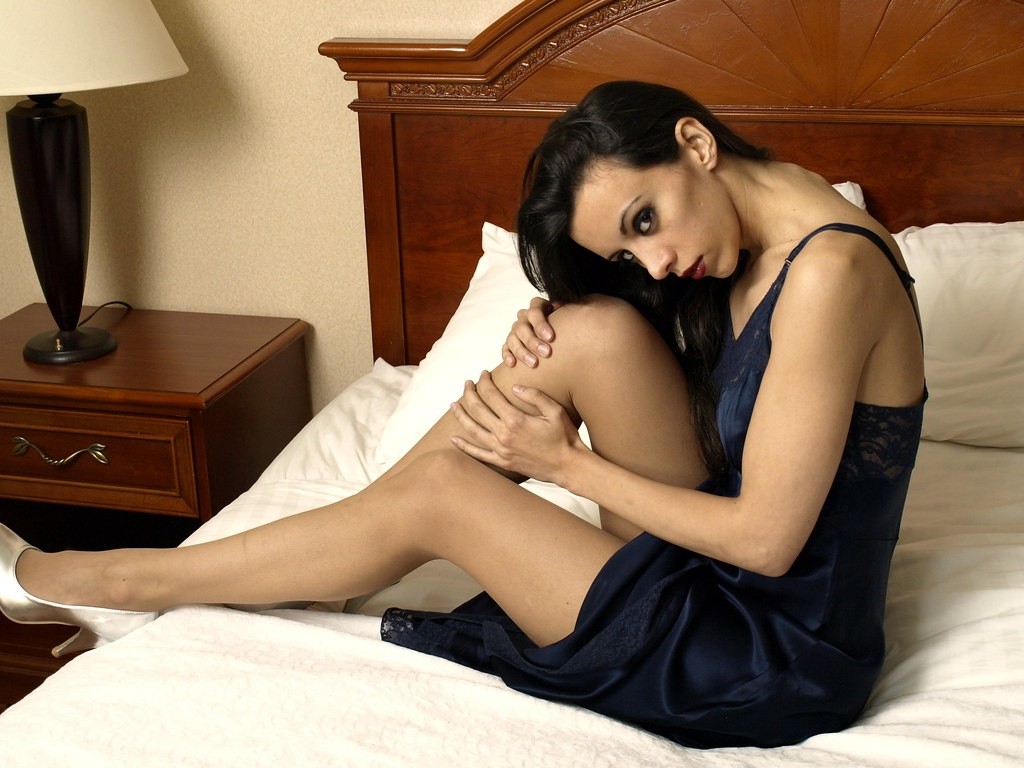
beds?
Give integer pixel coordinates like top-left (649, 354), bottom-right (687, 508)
top-left (0, 0), bottom-right (1024, 768)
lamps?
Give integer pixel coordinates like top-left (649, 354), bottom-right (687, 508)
top-left (0, 0), bottom-right (189, 368)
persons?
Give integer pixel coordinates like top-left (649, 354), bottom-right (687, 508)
top-left (0, 79), bottom-right (928, 751)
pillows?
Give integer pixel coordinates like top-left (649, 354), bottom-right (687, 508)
top-left (373, 179), bottom-right (868, 490)
top-left (888, 221), bottom-right (1024, 449)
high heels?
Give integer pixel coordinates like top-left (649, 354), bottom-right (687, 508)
top-left (0, 521), bottom-right (159, 658)
top-left (224, 580), bottom-right (402, 615)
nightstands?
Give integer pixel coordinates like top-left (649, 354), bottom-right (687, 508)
top-left (0, 303), bottom-right (312, 714)
